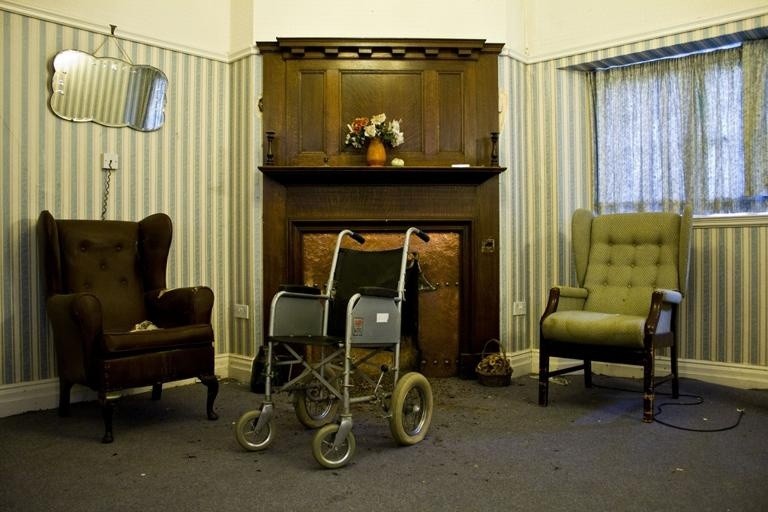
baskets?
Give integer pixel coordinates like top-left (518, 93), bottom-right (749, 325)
top-left (476, 339), bottom-right (513, 387)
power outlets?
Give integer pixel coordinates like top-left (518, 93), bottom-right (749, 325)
top-left (103, 152), bottom-right (119, 169)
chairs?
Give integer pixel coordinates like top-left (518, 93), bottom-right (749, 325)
top-left (539, 202), bottom-right (693, 423)
top-left (36, 209), bottom-right (219, 444)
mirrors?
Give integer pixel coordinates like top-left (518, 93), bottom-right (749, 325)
top-left (48, 47), bottom-right (168, 132)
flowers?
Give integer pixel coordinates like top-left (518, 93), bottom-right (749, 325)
top-left (478, 352), bottom-right (511, 374)
top-left (344, 113), bottom-right (404, 149)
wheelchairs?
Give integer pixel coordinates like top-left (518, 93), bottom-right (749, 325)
top-left (236, 226), bottom-right (434, 472)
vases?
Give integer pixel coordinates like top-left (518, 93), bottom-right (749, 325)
top-left (367, 137), bottom-right (387, 166)
top-left (475, 369), bottom-right (513, 386)
